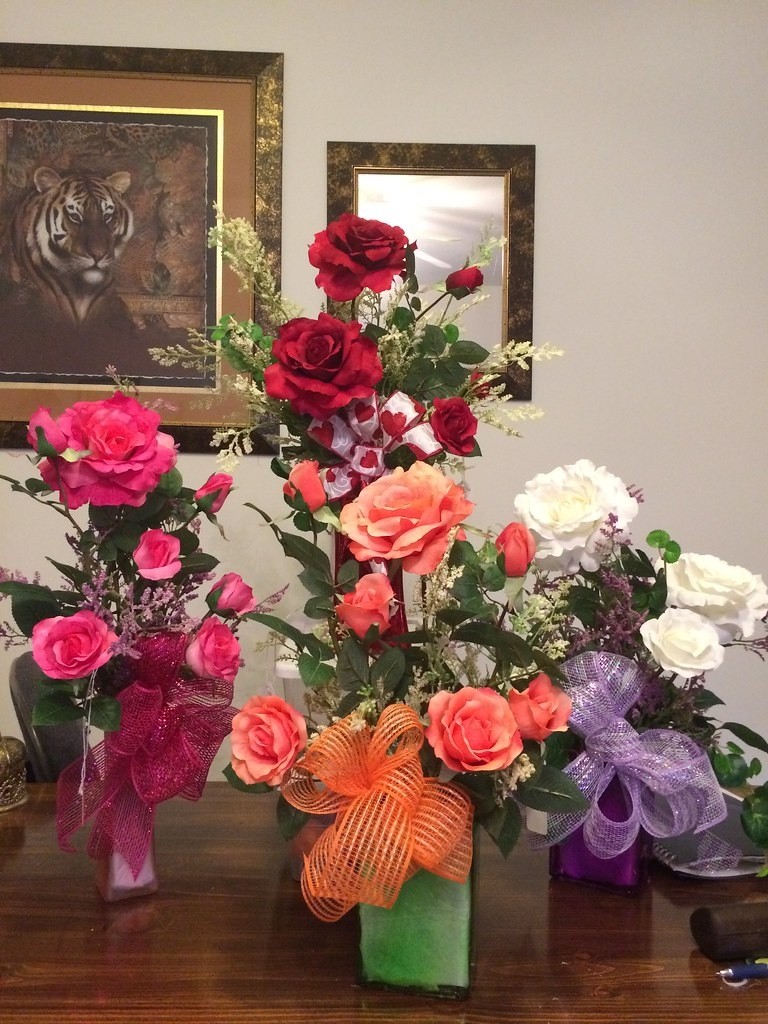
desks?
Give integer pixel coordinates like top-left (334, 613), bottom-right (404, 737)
top-left (0, 781), bottom-right (768, 1024)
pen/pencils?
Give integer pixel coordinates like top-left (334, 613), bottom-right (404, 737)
top-left (716, 963), bottom-right (768, 978)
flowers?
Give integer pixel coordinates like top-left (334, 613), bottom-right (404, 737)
top-left (0, 213), bottom-right (768, 881)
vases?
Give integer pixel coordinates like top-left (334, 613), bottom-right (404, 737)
top-left (545, 734), bottom-right (650, 893)
top-left (92, 743), bottom-right (158, 901)
top-left (358, 825), bottom-right (475, 1005)
top-left (287, 778), bottom-right (331, 886)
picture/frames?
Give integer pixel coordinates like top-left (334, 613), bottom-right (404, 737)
top-left (0, 41), bottom-right (284, 455)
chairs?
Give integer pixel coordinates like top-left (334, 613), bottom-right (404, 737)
top-left (9, 651), bottom-right (85, 784)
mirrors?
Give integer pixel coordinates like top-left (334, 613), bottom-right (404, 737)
top-left (326, 142), bottom-right (536, 404)
